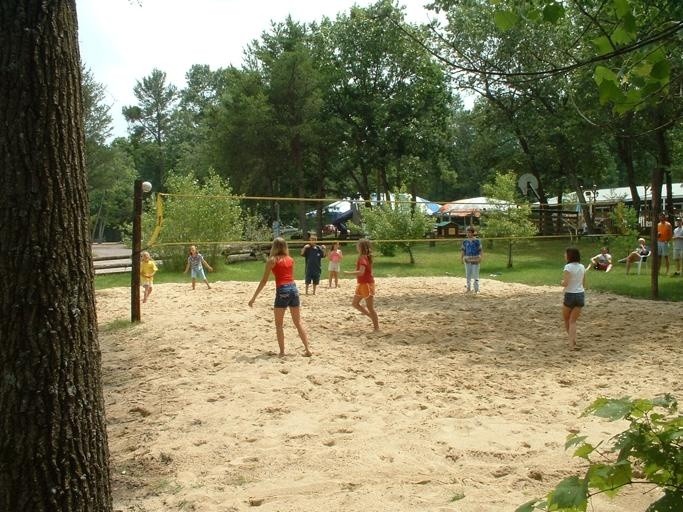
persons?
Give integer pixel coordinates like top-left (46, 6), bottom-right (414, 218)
top-left (617, 237), bottom-right (649, 278)
top-left (584, 247), bottom-right (613, 274)
top-left (342, 238), bottom-right (381, 335)
top-left (558, 247), bottom-right (586, 346)
top-left (140, 250), bottom-right (158, 304)
top-left (298, 234), bottom-right (326, 296)
top-left (460, 225), bottom-right (484, 295)
top-left (656, 212), bottom-right (673, 276)
top-left (181, 245), bottom-right (214, 291)
top-left (326, 241), bottom-right (343, 289)
top-left (247, 236), bottom-right (312, 358)
top-left (669, 217), bottom-right (682, 277)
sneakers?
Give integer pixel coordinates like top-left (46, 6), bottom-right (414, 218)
top-left (618, 258), bottom-right (626, 263)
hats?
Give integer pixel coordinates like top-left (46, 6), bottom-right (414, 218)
top-left (676, 218), bottom-right (683, 221)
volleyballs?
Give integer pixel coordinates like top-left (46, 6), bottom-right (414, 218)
top-left (141, 182), bottom-right (151, 193)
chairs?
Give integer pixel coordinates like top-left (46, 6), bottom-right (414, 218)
top-left (633, 250), bottom-right (652, 275)
